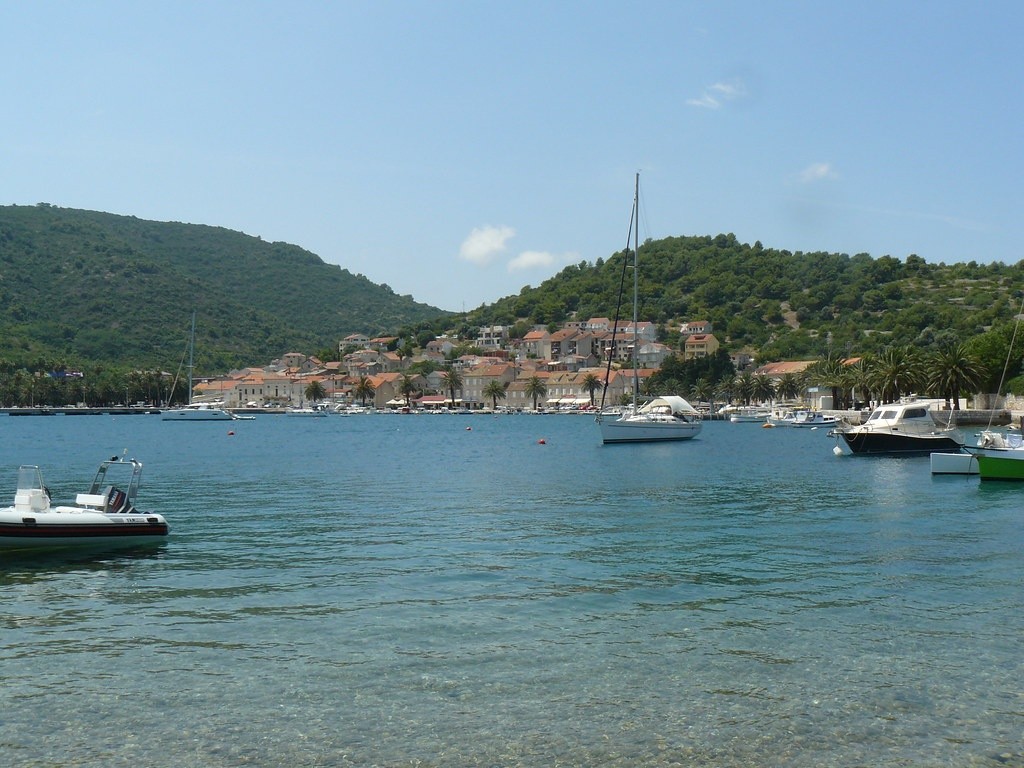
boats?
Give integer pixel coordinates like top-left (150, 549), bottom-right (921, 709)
top-left (826, 397), bottom-right (967, 456)
top-left (729, 404), bottom-right (843, 428)
top-left (0, 456), bottom-right (172, 552)
top-left (964, 445), bottom-right (1024, 480)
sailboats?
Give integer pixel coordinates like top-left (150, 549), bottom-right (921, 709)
top-left (159, 312), bottom-right (257, 422)
top-left (596, 172), bottom-right (704, 444)
